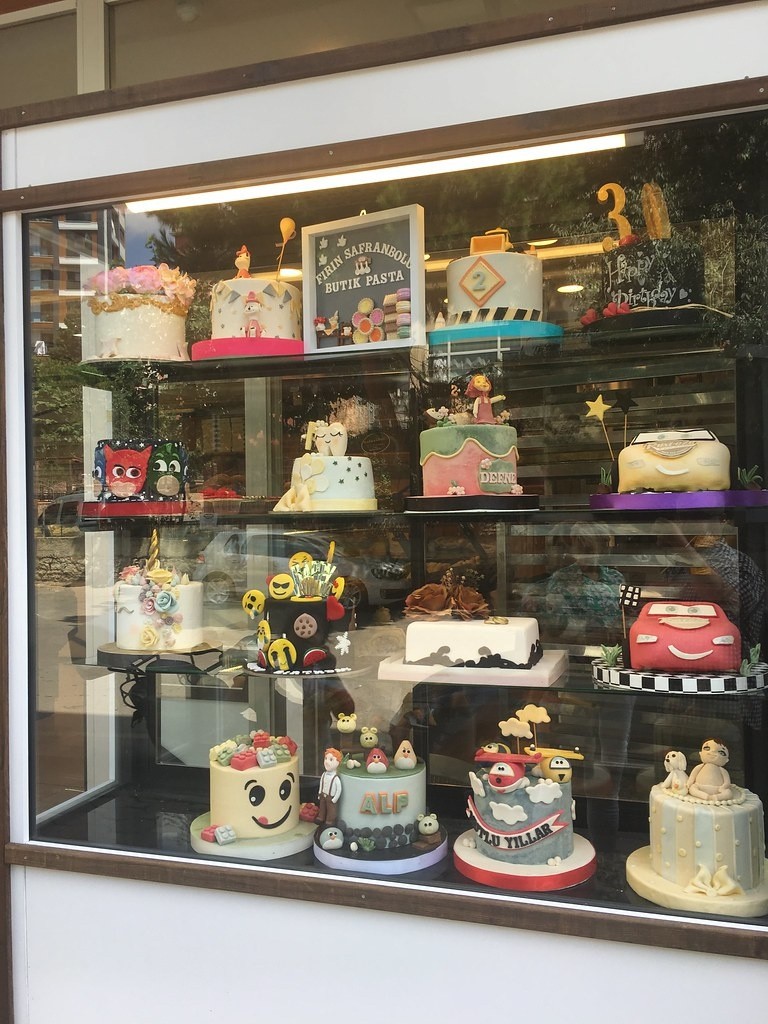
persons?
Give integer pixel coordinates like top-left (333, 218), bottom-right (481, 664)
top-left (233, 245), bottom-right (251, 280)
top-left (315, 748), bottom-right (342, 827)
top-left (245, 291), bottom-right (263, 338)
top-left (687, 737), bottom-right (733, 802)
top-left (464, 374), bottom-right (507, 425)
top-left (335, 512), bottom-right (768, 850)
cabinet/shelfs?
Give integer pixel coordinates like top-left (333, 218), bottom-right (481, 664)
top-left (23, 316), bottom-right (768, 955)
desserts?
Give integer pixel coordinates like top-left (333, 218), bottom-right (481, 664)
top-left (351, 287), bottom-right (411, 343)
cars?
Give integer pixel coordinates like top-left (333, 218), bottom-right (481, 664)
top-left (188, 527), bottom-right (409, 613)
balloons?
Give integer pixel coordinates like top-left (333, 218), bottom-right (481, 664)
top-left (279, 217), bottom-right (295, 243)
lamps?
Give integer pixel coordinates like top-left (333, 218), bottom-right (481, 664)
top-left (112, 129), bottom-right (651, 215)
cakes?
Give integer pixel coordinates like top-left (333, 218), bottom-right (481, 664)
top-left (201, 730), bottom-right (320, 845)
top-left (402, 583), bottom-right (543, 671)
top-left (600, 582), bottom-right (761, 677)
top-left (462, 704), bottom-right (584, 867)
top-left (112, 529), bottom-right (204, 651)
top-left (578, 182), bottom-right (707, 325)
top-left (445, 229), bottom-right (544, 323)
top-left (92, 436), bottom-right (190, 502)
top-left (647, 736), bottom-right (766, 897)
top-left (79, 263), bottom-right (196, 362)
top-left (210, 216), bottom-right (302, 340)
top-left (585, 388), bottom-right (764, 494)
top-left (418, 374), bottom-right (524, 496)
top-left (272, 420), bottom-right (378, 511)
top-left (241, 539), bottom-right (357, 675)
top-left (314, 712), bottom-right (441, 852)
